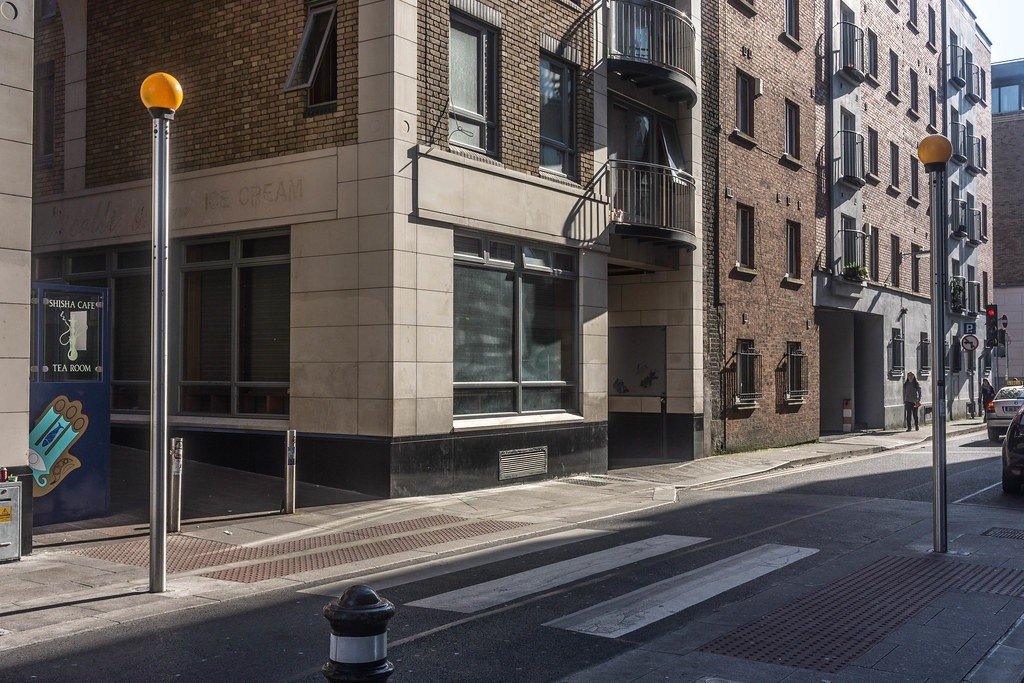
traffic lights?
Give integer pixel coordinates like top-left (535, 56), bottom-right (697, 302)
top-left (985, 304), bottom-right (999, 347)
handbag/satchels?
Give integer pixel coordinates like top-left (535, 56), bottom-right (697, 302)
top-left (914, 400), bottom-right (920, 408)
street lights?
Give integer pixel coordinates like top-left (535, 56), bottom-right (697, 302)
top-left (919, 135), bottom-right (955, 553)
top-left (140, 72), bottom-right (184, 592)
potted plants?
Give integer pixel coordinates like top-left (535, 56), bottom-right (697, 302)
top-left (842, 260), bottom-right (869, 280)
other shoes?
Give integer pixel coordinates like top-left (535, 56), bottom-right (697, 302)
top-left (916, 426), bottom-right (918, 431)
top-left (906, 428), bottom-right (911, 432)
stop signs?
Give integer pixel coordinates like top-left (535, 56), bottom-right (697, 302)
top-left (1002, 315), bottom-right (1008, 327)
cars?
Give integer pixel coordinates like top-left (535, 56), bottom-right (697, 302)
top-left (986, 378), bottom-right (1024, 441)
top-left (1002, 403), bottom-right (1024, 495)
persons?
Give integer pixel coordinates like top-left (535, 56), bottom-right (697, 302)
top-left (904, 371), bottom-right (922, 432)
top-left (978, 379), bottom-right (995, 423)
